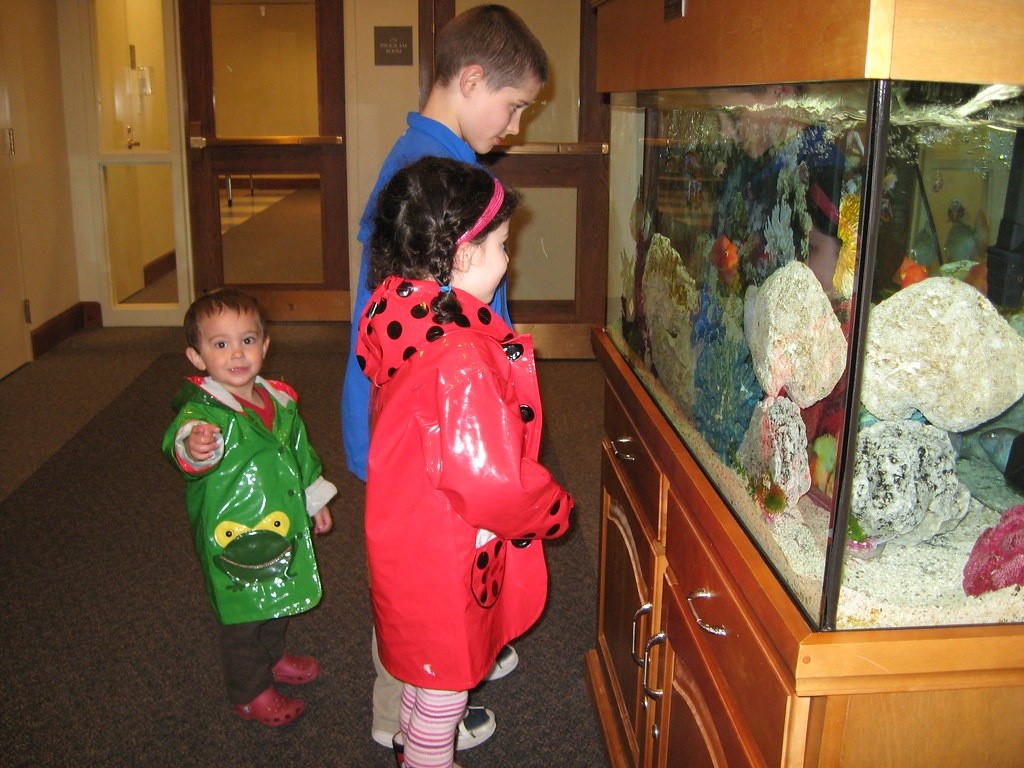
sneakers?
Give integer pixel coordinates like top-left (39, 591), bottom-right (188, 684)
top-left (372, 705), bottom-right (497, 751)
top-left (482, 643), bottom-right (518, 682)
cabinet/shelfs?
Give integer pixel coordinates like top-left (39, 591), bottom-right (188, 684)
top-left (584, 322), bottom-right (1024, 768)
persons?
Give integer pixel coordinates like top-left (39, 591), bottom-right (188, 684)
top-left (162, 287), bottom-right (338, 726)
top-left (356, 158), bottom-right (573, 768)
top-left (341, 5), bottom-right (548, 751)
top-left (692, 80), bottom-right (857, 469)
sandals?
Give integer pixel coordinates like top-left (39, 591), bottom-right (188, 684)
top-left (234, 685), bottom-right (306, 725)
top-left (270, 652), bottom-right (320, 684)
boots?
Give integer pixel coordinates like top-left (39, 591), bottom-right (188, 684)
top-left (392, 730), bottom-right (463, 768)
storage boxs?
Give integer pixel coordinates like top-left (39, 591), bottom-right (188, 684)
top-left (605, 78), bottom-right (1024, 632)
